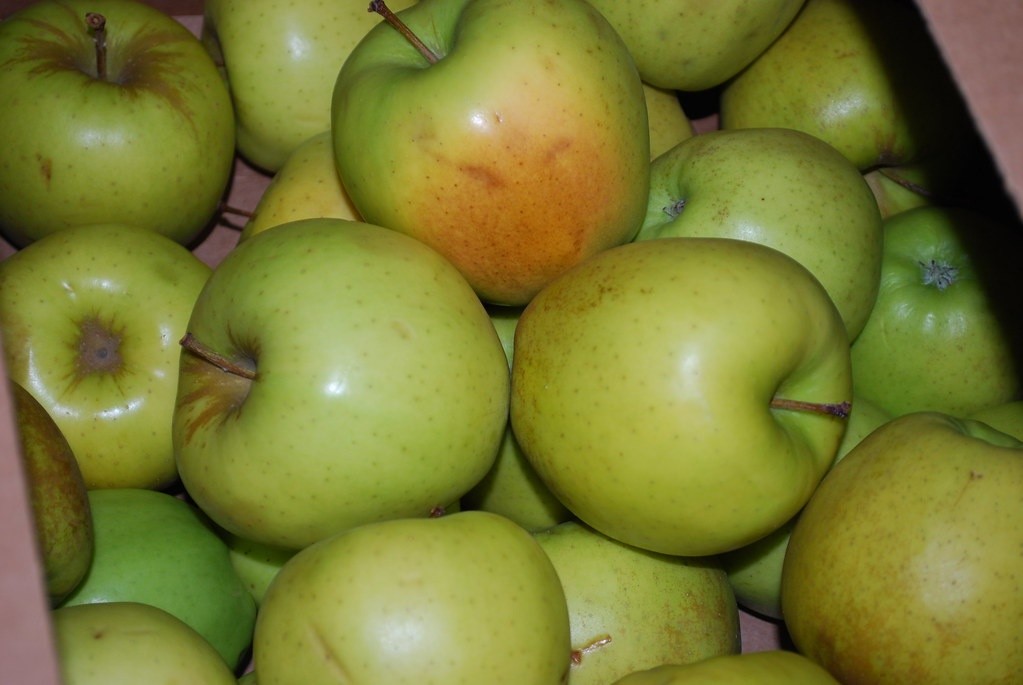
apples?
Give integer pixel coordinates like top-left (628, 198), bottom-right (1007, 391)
top-left (0, 0), bottom-right (1022, 685)
